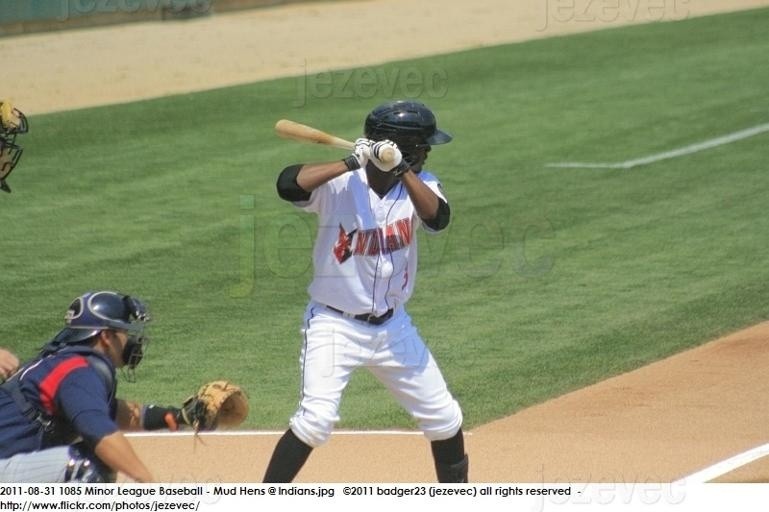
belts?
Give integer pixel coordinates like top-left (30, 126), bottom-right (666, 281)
top-left (326, 305), bottom-right (393, 325)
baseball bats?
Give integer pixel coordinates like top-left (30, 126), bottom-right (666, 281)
top-left (275, 120), bottom-right (395, 164)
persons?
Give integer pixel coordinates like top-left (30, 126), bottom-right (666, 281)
top-left (0, 97), bottom-right (28, 382)
top-left (263, 101), bottom-right (470, 483)
top-left (0, 291), bottom-right (246, 484)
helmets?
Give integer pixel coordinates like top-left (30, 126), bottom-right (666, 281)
top-left (365, 99), bottom-right (453, 149)
top-left (53, 290), bottom-right (152, 384)
top-left (1, 101), bottom-right (28, 193)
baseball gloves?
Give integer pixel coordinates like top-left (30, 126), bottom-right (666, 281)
top-left (183, 380), bottom-right (249, 430)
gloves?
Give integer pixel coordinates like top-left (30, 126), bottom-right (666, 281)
top-left (343, 138), bottom-right (376, 171)
top-left (370, 139), bottom-right (411, 177)
